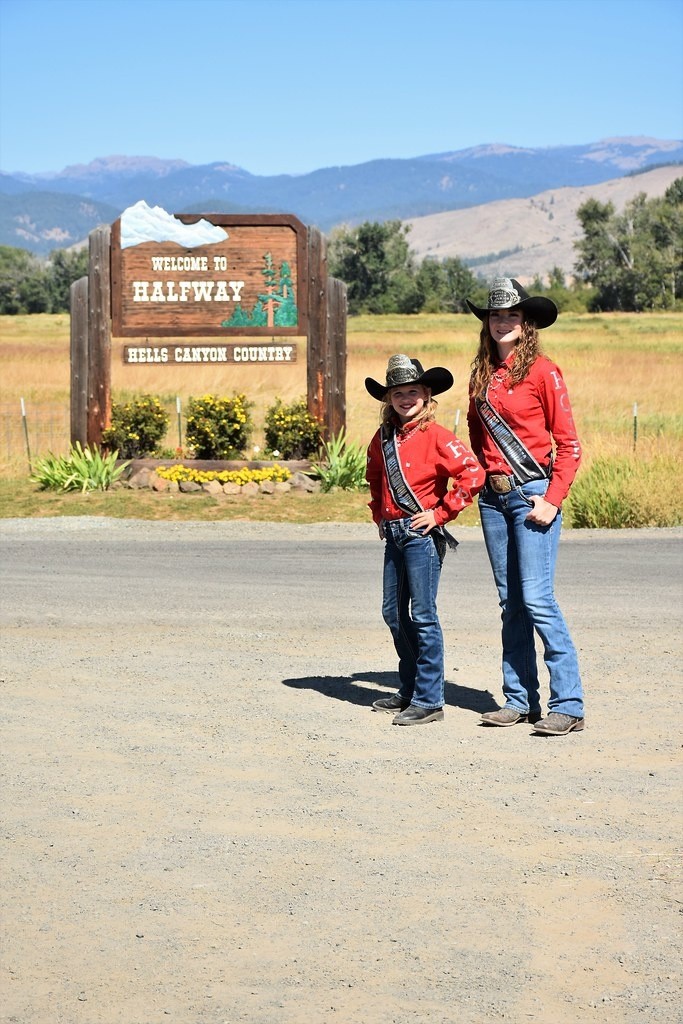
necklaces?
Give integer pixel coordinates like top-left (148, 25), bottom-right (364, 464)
top-left (395, 423), bottom-right (422, 443)
top-left (489, 369), bottom-right (511, 391)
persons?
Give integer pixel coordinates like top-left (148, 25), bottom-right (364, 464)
top-left (467, 276), bottom-right (585, 735)
top-left (365, 354), bottom-right (486, 726)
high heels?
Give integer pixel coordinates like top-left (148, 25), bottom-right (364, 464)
top-left (532, 712), bottom-right (585, 734)
top-left (393, 704), bottom-right (445, 724)
top-left (372, 695), bottom-right (410, 712)
top-left (479, 708), bottom-right (541, 726)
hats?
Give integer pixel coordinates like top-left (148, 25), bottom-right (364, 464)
top-left (365, 354), bottom-right (454, 403)
top-left (465, 277), bottom-right (557, 330)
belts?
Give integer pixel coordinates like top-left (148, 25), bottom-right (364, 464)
top-left (482, 467), bottom-right (551, 495)
